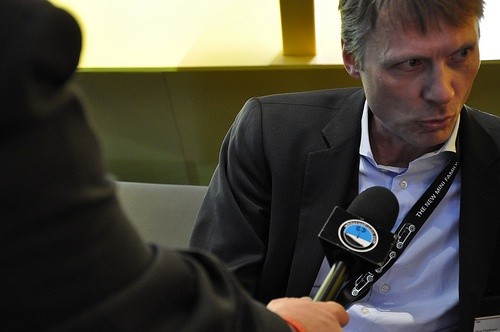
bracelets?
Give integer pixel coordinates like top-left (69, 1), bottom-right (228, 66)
top-left (278, 314), bottom-right (306, 332)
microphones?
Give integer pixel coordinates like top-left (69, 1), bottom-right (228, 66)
top-left (312, 186), bottom-right (399, 301)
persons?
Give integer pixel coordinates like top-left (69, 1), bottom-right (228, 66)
top-left (186, 0), bottom-right (500, 332)
top-left (0, 0), bottom-right (351, 332)
